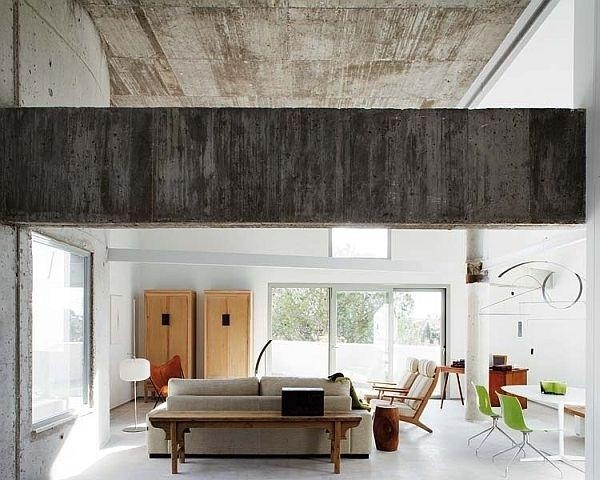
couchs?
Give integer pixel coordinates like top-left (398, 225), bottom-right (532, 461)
top-left (145, 376), bottom-right (371, 459)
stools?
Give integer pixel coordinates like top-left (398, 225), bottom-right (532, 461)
top-left (373, 405), bottom-right (400, 451)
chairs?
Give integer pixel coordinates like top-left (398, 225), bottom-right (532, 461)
top-left (466, 381), bottom-right (528, 458)
top-left (369, 360), bottom-right (438, 435)
top-left (493, 390), bottom-right (563, 478)
top-left (364, 357), bottom-right (420, 402)
top-left (145, 356), bottom-right (185, 407)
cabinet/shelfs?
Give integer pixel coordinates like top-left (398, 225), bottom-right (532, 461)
top-left (144, 290), bottom-right (197, 400)
top-left (203, 291), bottom-right (251, 380)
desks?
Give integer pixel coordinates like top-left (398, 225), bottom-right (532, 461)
top-left (149, 407), bottom-right (362, 476)
top-left (500, 383), bottom-right (586, 476)
top-left (438, 363), bottom-right (529, 409)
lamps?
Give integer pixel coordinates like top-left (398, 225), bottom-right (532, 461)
top-left (118, 357), bottom-right (151, 433)
